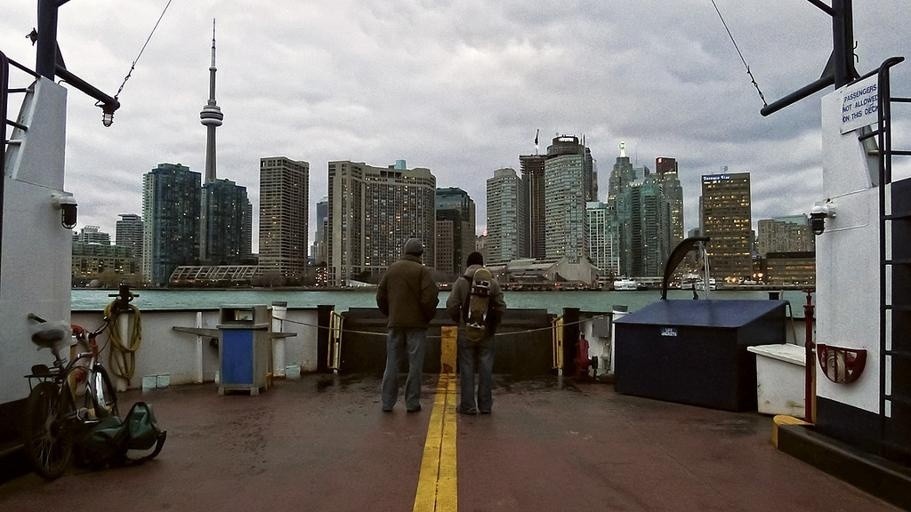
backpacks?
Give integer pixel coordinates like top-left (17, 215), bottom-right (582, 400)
top-left (461, 273), bottom-right (501, 337)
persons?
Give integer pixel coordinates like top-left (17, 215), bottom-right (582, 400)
top-left (373, 235), bottom-right (439, 411)
top-left (445, 250), bottom-right (507, 413)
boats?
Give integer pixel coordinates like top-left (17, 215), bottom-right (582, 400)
top-left (614, 279), bottom-right (648, 291)
top-left (681, 274), bottom-right (717, 290)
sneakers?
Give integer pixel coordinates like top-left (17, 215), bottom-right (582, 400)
top-left (459, 406), bottom-right (477, 416)
top-left (382, 405), bottom-right (394, 412)
top-left (481, 407), bottom-right (492, 414)
top-left (407, 403), bottom-right (421, 413)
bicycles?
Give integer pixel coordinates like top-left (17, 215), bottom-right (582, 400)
top-left (24, 311), bottom-right (122, 477)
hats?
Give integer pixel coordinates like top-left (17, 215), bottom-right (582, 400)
top-left (404, 238), bottom-right (424, 254)
top-left (467, 252), bottom-right (483, 267)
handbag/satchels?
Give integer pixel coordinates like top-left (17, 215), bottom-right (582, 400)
top-left (83, 400), bottom-right (167, 470)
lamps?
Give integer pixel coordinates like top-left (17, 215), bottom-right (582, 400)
top-left (53, 194), bottom-right (77, 229)
top-left (809, 205), bottom-right (836, 235)
top-left (102, 111), bottom-right (114, 126)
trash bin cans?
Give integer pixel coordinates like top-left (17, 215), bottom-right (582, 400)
top-left (216, 304), bottom-right (274, 397)
